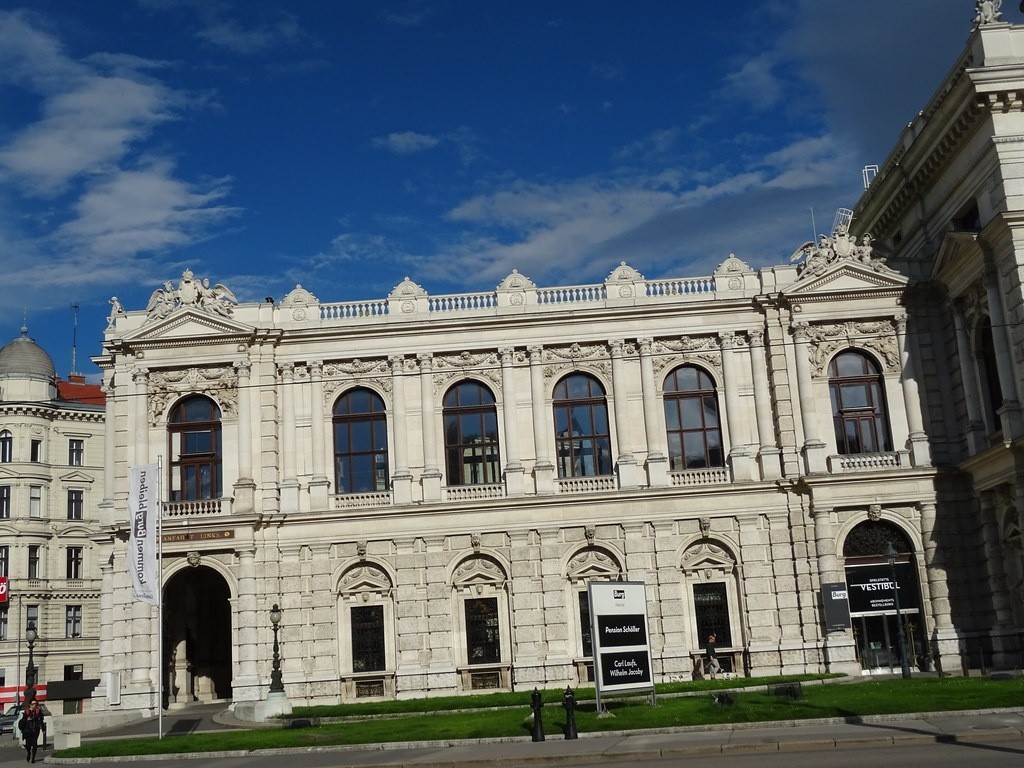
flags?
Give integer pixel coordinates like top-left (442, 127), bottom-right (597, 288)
top-left (127, 462), bottom-right (159, 606)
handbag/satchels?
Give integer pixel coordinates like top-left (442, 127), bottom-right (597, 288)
top-left (18, 710), bottom-right (27, 731)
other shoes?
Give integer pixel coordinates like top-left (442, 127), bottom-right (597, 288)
top-left (31, 756), bottom-right (35, 764)
top-left (26, 752), bottom-right (31, 763)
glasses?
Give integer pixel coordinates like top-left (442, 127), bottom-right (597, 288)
top-left (31, 703), bottom-right (37, 706)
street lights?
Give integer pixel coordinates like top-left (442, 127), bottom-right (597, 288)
top-left (267, 604), bottom-right (286, 693)
top-left (881, 541), bottom-right (911, 679)
top-left (21, 620), bottom-right (37, 706)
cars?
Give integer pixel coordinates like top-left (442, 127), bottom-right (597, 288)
top-left (0, 704), bottom-right (52, 736)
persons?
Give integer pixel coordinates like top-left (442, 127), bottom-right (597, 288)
top-left (18, 700), bottom-right (45, 763)
top-left (105, 296), bottom-right (120, 329)
top-left (793, 219), bottom-right (900, 281)
top-left (706, 633), bottom-right (719, 679)
top-left (140, 268), bottom-right (232, 326)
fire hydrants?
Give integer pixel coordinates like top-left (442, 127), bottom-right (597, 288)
top-left (530, 686), bottom-right (544, 742)
top-left (561, 685), bottom-right (578, 739)
